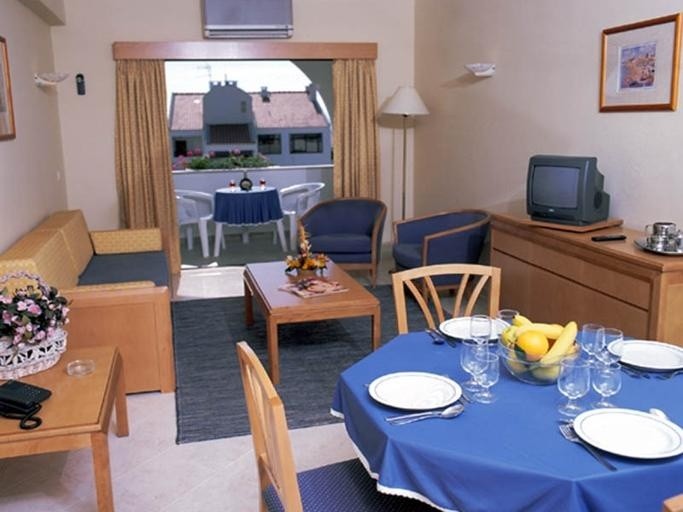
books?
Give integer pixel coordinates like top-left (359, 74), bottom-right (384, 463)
top-left (288, 277), bottom-right (349, 299)
top-left (277, 279), bottom-right (349, 293)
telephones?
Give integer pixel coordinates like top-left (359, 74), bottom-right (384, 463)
top-left (0, 377), bottom-right (52, 430)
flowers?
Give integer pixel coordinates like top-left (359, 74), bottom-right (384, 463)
top-left (284, 221), bottom-right (329, 274)
top-left (0, 284), bottom-right (74, 352)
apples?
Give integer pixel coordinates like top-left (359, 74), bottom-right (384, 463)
top-left (532, 365), bottom-right (558, 381)
top-left (502, 326), bottom-right (517, 344)
top-left (506, 351), bottom-right (526, 373)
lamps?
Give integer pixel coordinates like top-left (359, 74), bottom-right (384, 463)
top-left (380, 86), bottom-right (431, 224)
top-left (465, 62), bottom-right (495, 77)
top-left (34, 71), bottom-right (71, 88)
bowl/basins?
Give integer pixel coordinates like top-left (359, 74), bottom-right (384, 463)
top-left (498, 346), bottom-right (585, 387)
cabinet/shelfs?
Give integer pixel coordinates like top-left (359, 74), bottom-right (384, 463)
top-left (488, 211), bottom-right (683, 348)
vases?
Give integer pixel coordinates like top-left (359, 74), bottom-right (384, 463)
top-left (297, 267), bottom-right (317, 276)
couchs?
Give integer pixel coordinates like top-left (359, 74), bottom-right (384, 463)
top-left (0, 209), bottom-right (176, 394)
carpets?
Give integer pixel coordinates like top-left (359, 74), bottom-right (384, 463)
top-left (170, 284), bottom-right (454, 445)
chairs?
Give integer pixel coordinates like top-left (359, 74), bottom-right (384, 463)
top-left (272, 182), bottom-right (326, 251)
top-left (389, 264), bottom-right (502, 336)
top-left (236, 339), bottom-right (438, 511)
top-left (391, 207), bottom-right (492, 309)
top-left (174, 188), bottom-right (225, 258)
top-left (296, 196), bottom-right (388, 288)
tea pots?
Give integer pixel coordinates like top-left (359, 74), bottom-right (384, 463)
top-left (645, 222), bottom-right (682, 240)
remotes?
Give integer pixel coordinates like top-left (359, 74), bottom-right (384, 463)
top-left (592, 233), bottom-right (628, 243)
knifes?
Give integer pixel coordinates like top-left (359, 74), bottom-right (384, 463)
top-left (390, 414), bottom-right (441, 424)
top-left (433, 328), bottom-right (457, 348)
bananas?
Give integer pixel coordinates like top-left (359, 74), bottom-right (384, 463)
top-left (540, 321), bottom-right (577, 368)
top-left (523, 322), bottom-right (562, 339)
top-left (512, 315), bottom-right (531, 326)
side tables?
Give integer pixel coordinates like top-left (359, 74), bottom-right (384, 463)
top-left (0, 346), bottom-right (129, 511)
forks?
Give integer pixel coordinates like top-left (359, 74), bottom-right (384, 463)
top-left (559, 423), bottom-right (619, 471)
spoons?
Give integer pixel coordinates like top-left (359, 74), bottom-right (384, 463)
top-left (424, 328), bottom-right (445, 345)
top-left (384, 404), bottom-right (465, 422)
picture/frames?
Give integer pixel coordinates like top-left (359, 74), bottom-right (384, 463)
top-left (0, 36), bottom-right (17, 140)
top-left (598, 11), bottom-right (683, 113)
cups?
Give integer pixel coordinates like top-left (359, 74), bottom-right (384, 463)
top-left (229, 178), bottom-right (235, 188)
top-left (666, 239), bottom-right (677, 251)
top-left (647, 236), bottom-right (668, 251)
top-left (259, 180), bottom-right (266, 188)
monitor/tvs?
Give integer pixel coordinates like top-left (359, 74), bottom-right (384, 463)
top-left (525, 153), bottom-right (610, 227)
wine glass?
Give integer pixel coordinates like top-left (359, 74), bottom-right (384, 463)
top-left (557, 358), bottom-right (592, 417)
top-left (591, 359), bottom-right (623, 409)
top-left (592, 327), bottom-right (628, 372)
top-left (580, 322), bottom-right (606, 358)
top-left (459, 341), bottom-right (490, 394)
top-left (500, 309), bottom-right (521, 322)
top-left (470, 314), bottom-right (491, 352)
top-left (470, 352), bottom-right (500, 405)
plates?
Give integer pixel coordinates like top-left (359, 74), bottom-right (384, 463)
top-left (571, 407), bottom-right (683, 460)
top-left (437, 316), bottom-right (512, 342)
top-left (367, 370), bottom-right (463, 411)
top-left (606, 338), bottom-right (683, 373)
